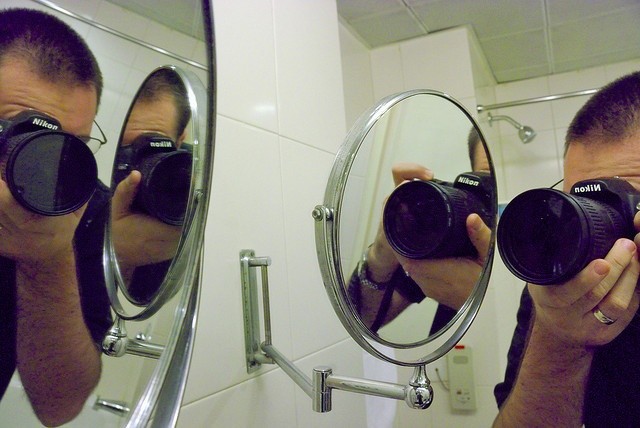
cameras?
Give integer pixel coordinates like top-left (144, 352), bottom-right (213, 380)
top-left (381, 170), bottom-right (497, 261)
top-left (495, 176), bottom-right (635, 285)
top-left (0, 109), bottom-right (99, 217)
top-left (113, 135), bottom-right (193, 225)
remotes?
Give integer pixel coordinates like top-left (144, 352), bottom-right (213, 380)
top-left (448, 345), bottom-right (478, 414)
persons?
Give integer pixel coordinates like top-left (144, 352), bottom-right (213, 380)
top-left (110, 65), bottom-right (194, 308)
top-left (0, 5), bottom-right (112, 428)
top-left (346, 125), bottom-right (495, 338)
top-left (491, 70), bottom-right (639, 428)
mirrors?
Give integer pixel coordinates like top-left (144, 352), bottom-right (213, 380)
top-left (106, 61), bottom-right (207, 357)
top-left (0, 0), bottom-right (217, 428)
top-left (238, 86), bottom-right (497, 415)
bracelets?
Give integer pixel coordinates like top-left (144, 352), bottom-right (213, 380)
top-left (358, 257), bottom-right (392, 290)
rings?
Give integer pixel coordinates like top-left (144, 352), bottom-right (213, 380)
top-left (405, 270), bottom-right (410, 277)
top-left (593, 308), bottom-right (618, 325)
